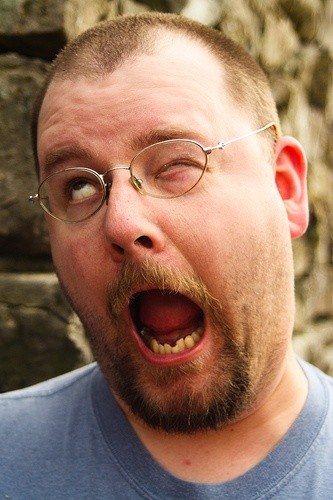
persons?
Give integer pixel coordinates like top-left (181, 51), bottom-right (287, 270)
top-left (2, 13), bottom-right (333, 499)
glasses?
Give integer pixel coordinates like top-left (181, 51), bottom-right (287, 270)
top-left (28, 121), bottom-right (280, 224)
